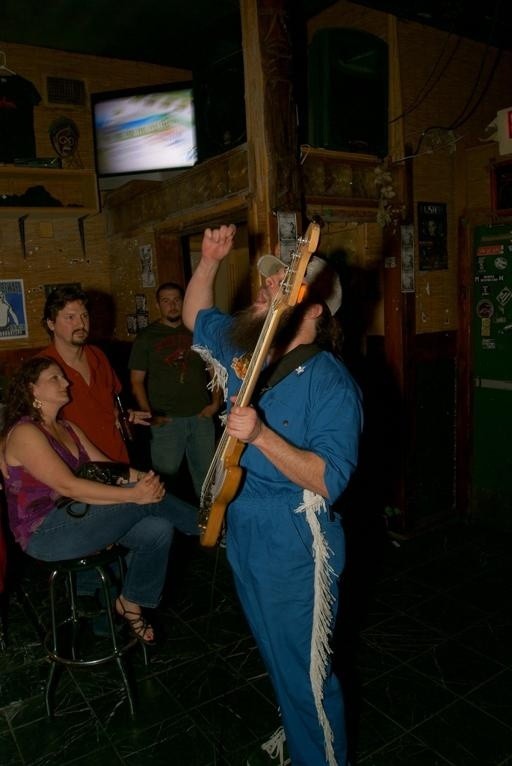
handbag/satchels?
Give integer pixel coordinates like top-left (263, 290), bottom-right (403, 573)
top-left (56, 462), bottom-right (129, 519)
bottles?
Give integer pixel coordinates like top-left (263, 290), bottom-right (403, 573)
top-left (115, 395), bottom-right (139, 445)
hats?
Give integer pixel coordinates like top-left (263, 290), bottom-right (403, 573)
top-left (256, 254), bottom-right (343, 317)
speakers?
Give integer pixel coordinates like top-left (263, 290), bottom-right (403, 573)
top-left (300, 25), bottom-right (390, 159)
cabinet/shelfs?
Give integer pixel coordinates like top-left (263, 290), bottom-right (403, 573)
top-left (0, 161), bottom-right (99, 217)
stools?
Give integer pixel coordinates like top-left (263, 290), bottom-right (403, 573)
top-left (42, 546), bottom-right (150, 715)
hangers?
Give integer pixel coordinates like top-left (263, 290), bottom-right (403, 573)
top-left (0, 49), bottom-right (18, 75)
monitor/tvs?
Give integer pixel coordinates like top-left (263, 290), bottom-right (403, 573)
top-left (89, 80), bottom-right (202, 177)
top-left (490, 159), bottom-right (512, 216)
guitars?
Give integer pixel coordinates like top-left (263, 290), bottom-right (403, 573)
top-left (199, 219), bottom-right (320, 549)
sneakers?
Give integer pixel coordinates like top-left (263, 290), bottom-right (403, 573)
top-left (246, 725), bottom-right (291, 766)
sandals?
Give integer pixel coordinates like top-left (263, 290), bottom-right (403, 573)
top-left (113, 596), bottom-right (156, 644)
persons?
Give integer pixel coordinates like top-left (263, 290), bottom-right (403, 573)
top-left (182, 203), bottom-right (367, 766)
top-left (127, 282), bottom-right (222, 499)
top-left (33, 287), bottom-right (154, 464)
top-left (428, 221), bottom-right (436, 236)
top-left (0, 357), bottom-right (204, 645)
